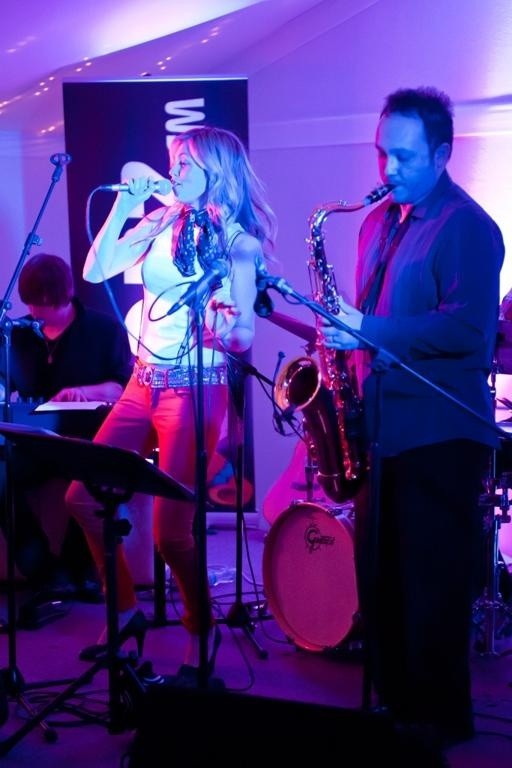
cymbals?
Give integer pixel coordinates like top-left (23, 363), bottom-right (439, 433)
top-left (266, 312), bottom-right (317, 345)
top-left (488, 319), bottom-right (512, 373)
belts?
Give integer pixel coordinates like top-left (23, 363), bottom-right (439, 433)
top-left (132, 359), bottom-right (227, 389)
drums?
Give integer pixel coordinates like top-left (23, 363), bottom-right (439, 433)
top-left (262, 501), bottom-right (361, 651)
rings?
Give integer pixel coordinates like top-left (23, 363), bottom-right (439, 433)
top-left (332, 335), bottom-right (334, 343)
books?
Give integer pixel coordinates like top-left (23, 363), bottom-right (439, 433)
top-left (28, 402), bottom-right (115, 413)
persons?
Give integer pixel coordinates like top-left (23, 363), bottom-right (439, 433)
top-left (313, 87), bottom-right (505, 756)
top-left (1, 253), bottom-right (134, 606)
top-left (64, 126), bottom-right (262, 692)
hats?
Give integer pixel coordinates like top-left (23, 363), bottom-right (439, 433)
top-left (19, 253), bottom-right (75, 305)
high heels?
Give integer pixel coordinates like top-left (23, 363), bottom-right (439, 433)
top-left (78, 608), bottom-right (147, 663)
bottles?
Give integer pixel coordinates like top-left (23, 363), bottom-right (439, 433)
top-left (207, 567), bottom-right (237, 588)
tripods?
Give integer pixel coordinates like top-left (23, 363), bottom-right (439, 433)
top-left (0, 166), bottom-right (94, 742)
top-left (165, 316), bottom-right (276, 660)
top-left (471, 445), bottom-right (512, 657)
top-left (0, 479), bottom-right (144, 768)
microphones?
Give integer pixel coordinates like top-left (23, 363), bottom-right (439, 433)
top-left (167, 261), bottom-right (227, 315)
top-left (253, 256), bottom-right (273, 318)
top-left (100, 179), bottom-right (171, 195)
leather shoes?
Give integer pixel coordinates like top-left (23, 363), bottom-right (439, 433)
top-left (176, 624), bottom-right (222, 679)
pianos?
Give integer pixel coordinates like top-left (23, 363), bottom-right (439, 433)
top-left (0, 396), bottom-right (111, 439)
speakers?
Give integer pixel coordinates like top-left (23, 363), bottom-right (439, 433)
top-left (129, 683), bottom-right (441, 768)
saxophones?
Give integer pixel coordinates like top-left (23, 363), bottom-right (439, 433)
top-left (275, 182), bottom-right (396, 501)
